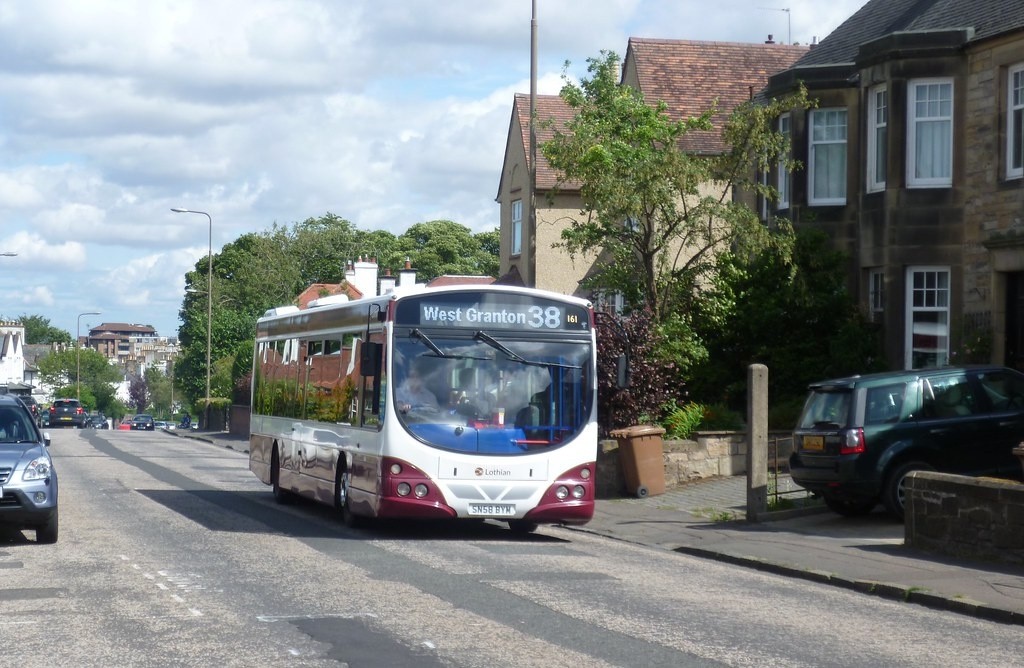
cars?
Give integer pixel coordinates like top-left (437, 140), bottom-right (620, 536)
top-left (85, 415), bottom-right (108, 429)
top-left (155, 422), bottom-right (166, 431)
top-left (132, 415), bottom-right (154, 430)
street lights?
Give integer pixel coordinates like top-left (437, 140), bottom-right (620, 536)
top-left (77, 312), bottom-right (101, 399)
top-left (170, 207), bottom-right (212, 429)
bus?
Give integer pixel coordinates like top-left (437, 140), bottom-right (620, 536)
top-left (250, 285), bottom-right (598, 533)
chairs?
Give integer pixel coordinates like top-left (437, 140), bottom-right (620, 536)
top-left (394, 386), bottom-right (497, 426)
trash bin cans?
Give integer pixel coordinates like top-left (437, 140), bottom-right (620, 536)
top-left (609, 424), bottom-right (666, 499)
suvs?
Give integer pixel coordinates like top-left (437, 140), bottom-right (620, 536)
top-left (0, 394), bottom-right (58, 544)
top-left (788, 366), bottom-right (1024, 524)
top-left (50, 399), bottom-right (84, 428)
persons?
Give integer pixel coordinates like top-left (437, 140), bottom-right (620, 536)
top-left (395, 366), bottom-right (442, 418)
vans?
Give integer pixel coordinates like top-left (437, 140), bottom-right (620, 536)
top-left (169, 424), bottom-right (175, 430)
